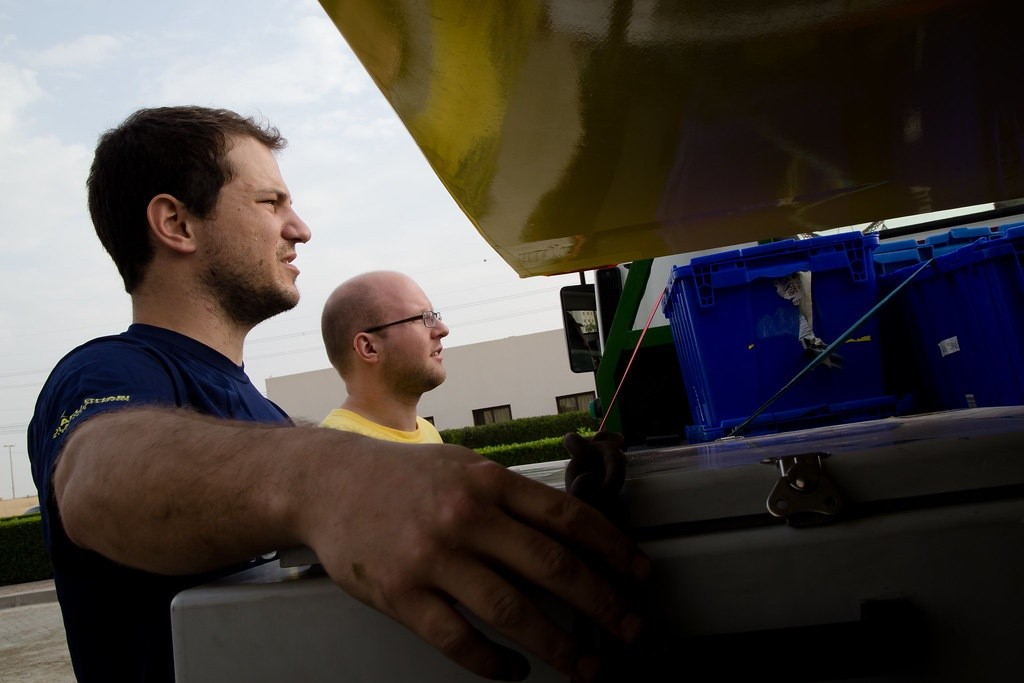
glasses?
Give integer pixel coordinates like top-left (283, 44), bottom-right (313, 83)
top-left (352, 311), bottom-right (443, 351)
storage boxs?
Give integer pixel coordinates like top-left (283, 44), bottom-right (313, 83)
top-left (657, 223), bottom-right (1024, 438)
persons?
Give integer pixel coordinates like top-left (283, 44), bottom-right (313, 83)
top-left (28, 107), bottom-right (651, 683)
top-left (318, 271), bottom-right (450, 444)
